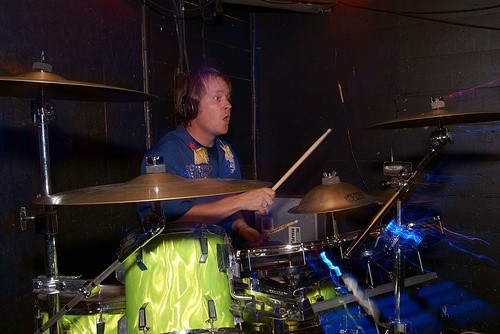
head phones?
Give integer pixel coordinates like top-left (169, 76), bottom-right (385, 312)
top-left (179, 76), bottom-right (198, 121)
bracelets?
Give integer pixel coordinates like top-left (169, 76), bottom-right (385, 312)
top-left (239, 224), bottom-right (249, 235)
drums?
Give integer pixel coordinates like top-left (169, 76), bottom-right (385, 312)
top-left (120, 222), bottom-right (239, 334)
top-left (307, 278), bottom-right (337, 304)
top-left (233, 277), bottom-right (320, 334)
top-left (33, 277), bottom-right (126, 334)
top-left (240, 241), bottom-right (309, 268)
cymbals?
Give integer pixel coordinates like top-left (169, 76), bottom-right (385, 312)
top-left (33, 174), bottom-right (273, 205)
top-left (287, 183), bottom-right (384, 215)
top-left (371, 109), bottom-right (499, 128)
top-left (363, 180), bottom-right (445, 204)
top-left (1, 73), bottom-right (156, 102)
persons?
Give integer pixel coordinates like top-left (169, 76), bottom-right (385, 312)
top-left (135, 67), bottom-right (286, 249)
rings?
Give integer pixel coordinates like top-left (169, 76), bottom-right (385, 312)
top-left (264, 203), bottom-right (268, 209)
